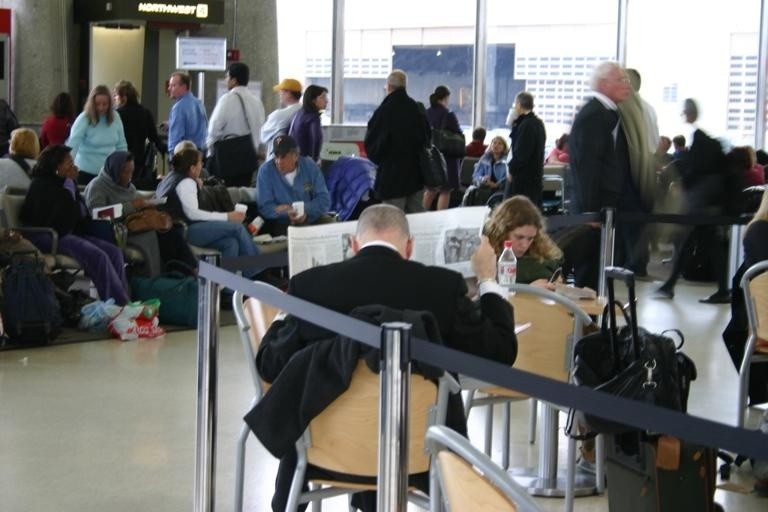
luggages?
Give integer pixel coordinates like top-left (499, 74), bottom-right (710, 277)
top-left (601, 265), bottom-right (726, 512)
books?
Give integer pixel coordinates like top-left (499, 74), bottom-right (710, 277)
top-left (275, 205), bottom-right (493, 303)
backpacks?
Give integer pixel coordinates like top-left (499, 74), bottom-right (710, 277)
top-left (198, 175), bottom-right (237, 213)
top-left (563, 299), bottom-right (697, 455)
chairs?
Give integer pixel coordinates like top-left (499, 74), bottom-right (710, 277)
top-left (458, 157), bottom-right (568, 217)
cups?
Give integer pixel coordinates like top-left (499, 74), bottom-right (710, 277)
top-left (292, 200), bottom-right (305, 217)
top-left (234, 203), bottom-right (247, 214)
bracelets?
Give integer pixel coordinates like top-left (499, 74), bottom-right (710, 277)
top-left (477, 277), bottom-right (501, 286)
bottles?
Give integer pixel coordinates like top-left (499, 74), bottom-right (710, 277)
top-left (497, 241), bottom-right (517, 296)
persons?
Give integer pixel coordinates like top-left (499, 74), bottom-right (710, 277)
top-left (724, 188), bottom-right (768, 403)
top-left (3, 62), bottom-right (330, 307)
top-left (485, 196), bottom-right (567, 294)
top-left (252, 204), bottom-right (519, 512)
top-left (361, 70), bottom-right (547, 217)
top-left (547, 62), bottom-right (768, 308)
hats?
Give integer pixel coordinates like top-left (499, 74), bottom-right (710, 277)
top-left (268, 133), bottom-right (298, 156)
top-left (272, 77), bottom-right (302, 93)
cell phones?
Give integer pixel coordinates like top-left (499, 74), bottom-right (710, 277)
top-left (549, 267), bottom-right (562, 283)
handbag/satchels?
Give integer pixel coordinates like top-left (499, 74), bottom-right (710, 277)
top-left (414, 125), bottom-right (466, 190)
top-left (0, 203), bottom-right (198, 350)
top-left (212, 131), bottom-right (261, 180)
